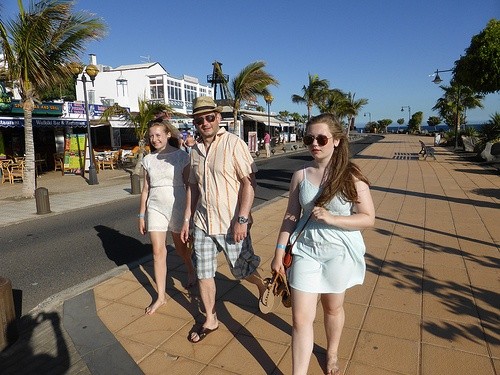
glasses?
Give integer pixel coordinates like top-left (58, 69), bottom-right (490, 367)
top-left (147, 118), bottom-right (163, 127)
top-left (302, 134), bottom-right (333, 146)
top-left (193, 114), bottom-right (219, 125)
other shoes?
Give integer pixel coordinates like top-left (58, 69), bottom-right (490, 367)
top-left (272, 270), bottom-right (292, 308)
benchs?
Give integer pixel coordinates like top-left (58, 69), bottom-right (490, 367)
top-left (419, 140), bottom-right (438, 160)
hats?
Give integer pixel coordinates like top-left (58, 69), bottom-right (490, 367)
top-left (186, 96), bottom-right (223, 118)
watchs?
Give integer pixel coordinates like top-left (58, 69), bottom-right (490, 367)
top-left (237, 216), bottom-right (248, 223)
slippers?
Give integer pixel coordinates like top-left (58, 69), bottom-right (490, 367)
top-left (187, 322), bottom-right (219, 343)
top-left (258, 277), bottom-right (272, 300)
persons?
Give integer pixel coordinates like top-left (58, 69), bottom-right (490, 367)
top-left (184, 131), bottom-right (195, 153)
top-left (271, 114), bottom-right (375, 375)
top-left (179, 134), bottom-right (184, 147)
top-left (263, 130), bottom-right (271, 158)
top-left (180, 96), bottom-right (271, 344)
top-left (138, 116), bottom-right (198, 314)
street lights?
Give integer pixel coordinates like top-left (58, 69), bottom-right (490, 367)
top-left (401, 105), bottom-right (411, 120)
top-left (81, 64), bottom-right (100, 185)
top-left (264, 95), bottom-right (274, 136)
top-left (433, 67), bottom-right (461, 148)
top-left (364, 112), bottom-right (371, 122)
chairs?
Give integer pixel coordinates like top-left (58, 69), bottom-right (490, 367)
top-left (93, 146), bottom-right (151, 174)
top-left (54, 153), bottom-right (63, 172)
top-left (2, 157), bottom-right (26, 184)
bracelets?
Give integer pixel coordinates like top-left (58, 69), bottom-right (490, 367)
top-left (183, 218), bottom-right (189, 221)
top-left (138, 214), bottom-right (145, 218)
top-left (276, 244), bottom-right (287, 250)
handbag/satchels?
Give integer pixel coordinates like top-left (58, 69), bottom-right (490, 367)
top-left (283, 243), bottom-right (293, 268)
